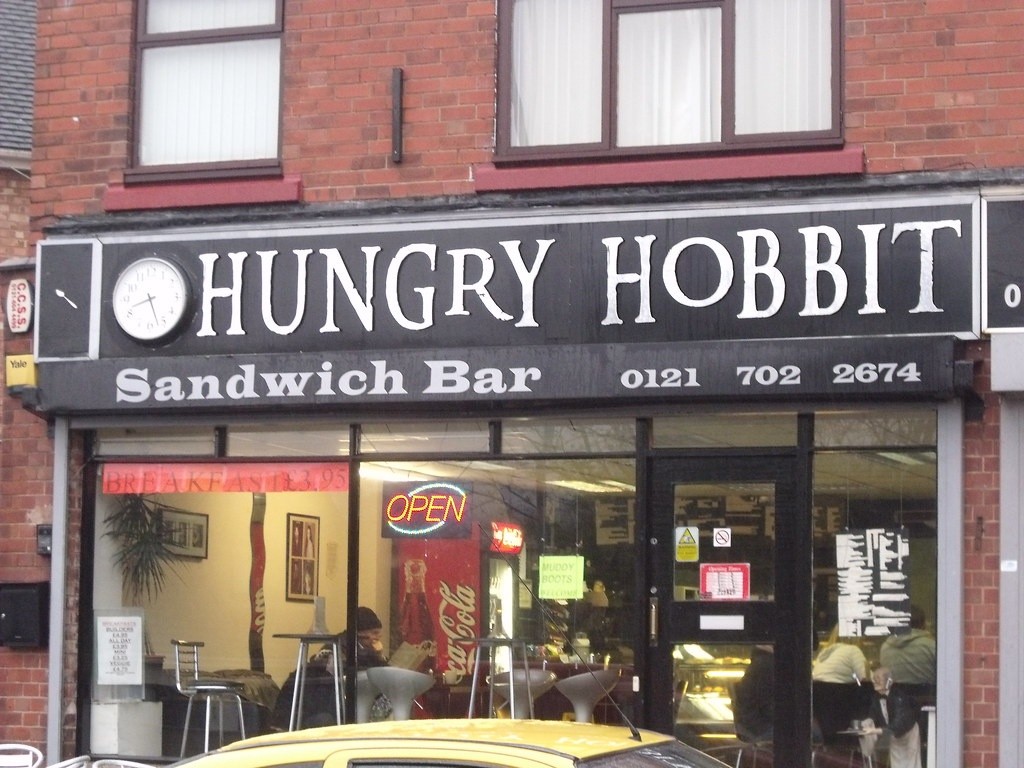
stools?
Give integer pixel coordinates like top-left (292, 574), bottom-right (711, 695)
top-left (340, 666), bottom-right (623, 724)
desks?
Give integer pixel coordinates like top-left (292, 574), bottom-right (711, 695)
top-left (271, 633), bottom-right (370, 731)
top-left (451, 637), bottom-right (543, 720)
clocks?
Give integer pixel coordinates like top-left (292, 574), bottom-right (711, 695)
top-left (112, 256), bottom-right (193, 345)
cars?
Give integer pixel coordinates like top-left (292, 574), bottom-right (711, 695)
top-left (145, 669), bottom-right (281, 751)
top-left (158, 718), bottom-right (731, 767)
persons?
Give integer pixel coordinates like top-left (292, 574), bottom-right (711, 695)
top-left (292, 563), bottom-right (300, 594)
top-left (270, 607), bottom-right (390, 731)
top-left (303, 526), bottom-right (314, 558)
top-left (810, 625), bottom-right (867, 682)
top-left (302, 563), bottom-right (313, 595)
top-left (292, 525), bottom-right (301, 556)
top-left (734, 629), bottom-right (824, 743)
top-left (878, 603), bottom-right (937, 685)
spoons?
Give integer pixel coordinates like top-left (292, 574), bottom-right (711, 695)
top-left (56, 289), bottom-right (78, 309)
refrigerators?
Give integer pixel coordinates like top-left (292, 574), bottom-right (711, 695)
top-left (397, 522), bottom-right (526, 719)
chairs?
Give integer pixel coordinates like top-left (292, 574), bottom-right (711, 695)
top-left (170, 638), bottom-right (249, 758)
top-left (730, 677), bottom-right (936, 767)
top-left (0, 742), bottom-right (155, 767)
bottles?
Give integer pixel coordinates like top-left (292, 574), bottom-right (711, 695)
top-left (400, 559), bottom-right (439, 719)
top-left (571, 632), bottom-right (590, 662)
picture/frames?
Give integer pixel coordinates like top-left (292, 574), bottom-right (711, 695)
top-left (156, 507), bottom-right (208, 558)
top-left (285, 512), bottom-right (320, 602)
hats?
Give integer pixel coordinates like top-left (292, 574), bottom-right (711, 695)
top-left (358, 606), bottom-right (382, 629)
